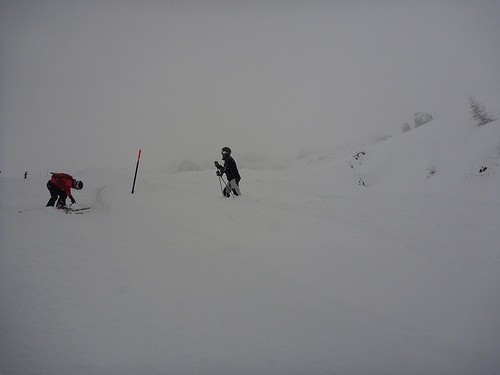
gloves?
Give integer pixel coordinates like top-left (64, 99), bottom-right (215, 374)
top-left (69, 195), bottom-right (75, 204)
top-left (214, 160), bottom-right (219, 168)
top-left (215, 171), bottom-right (221, 176)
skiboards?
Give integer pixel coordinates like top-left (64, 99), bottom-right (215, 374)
top-left (17, 198), bottom-right (90, 215)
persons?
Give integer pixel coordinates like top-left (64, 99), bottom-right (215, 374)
top-left (214, 147), bottom-right (242, 197)
top-left (46, 173), bottom-right (83, 209)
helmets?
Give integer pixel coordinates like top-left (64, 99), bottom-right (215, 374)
top-left (221, 147), bottom-right (231, 160)
top-left (74, 180), bottom-right (83, 190)
